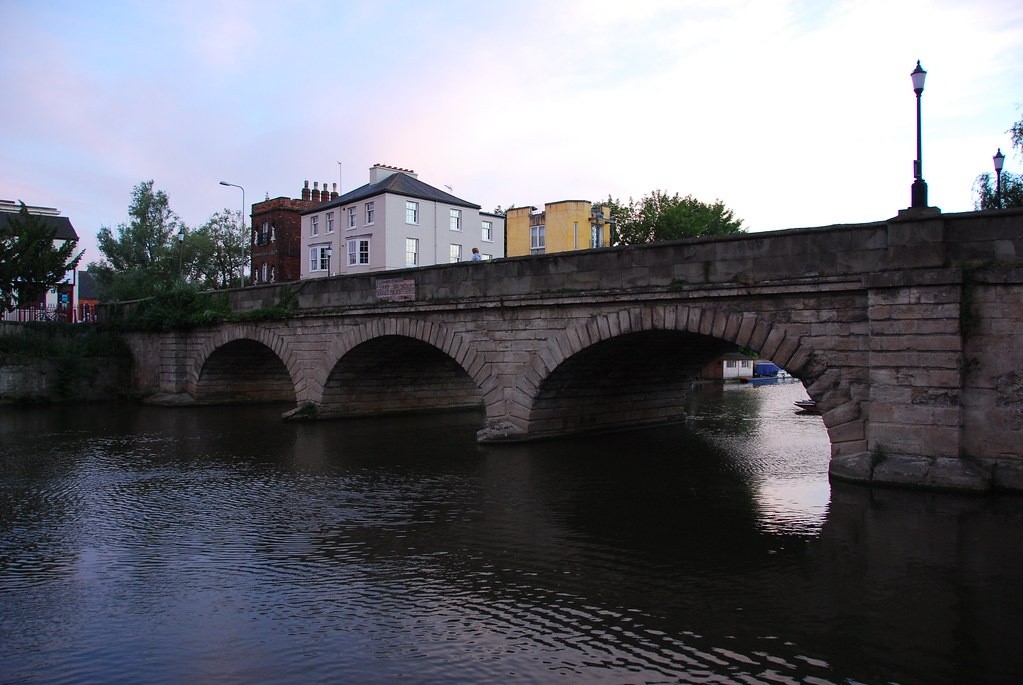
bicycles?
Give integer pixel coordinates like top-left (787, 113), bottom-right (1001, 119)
top-left (34, 307), bottom-right (68, 322)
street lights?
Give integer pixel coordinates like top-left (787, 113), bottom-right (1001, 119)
top-left (177, 227), bottom-right (185, 278)
top-left (326, 245), bottom-right (333, 277)
top-left (909, 57), bottom-right (928, 208)
top-left (218, 180), bottom-right (245, 288)
top-left (992, 147), bottom-right (1006, 211)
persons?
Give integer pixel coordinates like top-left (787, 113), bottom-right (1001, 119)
top-left (84, 302), bottom-right (91, 313)
top-left (270, 275), bottom-right (273, 280)
top-left (471, 248), bottom-right (481, 261)
top-left (252, 276), bottom-right (258, 285)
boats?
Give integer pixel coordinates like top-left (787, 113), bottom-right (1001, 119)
top-left (793, 398), bottom-right (820, 412)
top-left (740, 362), bottom-right (779, 384)
